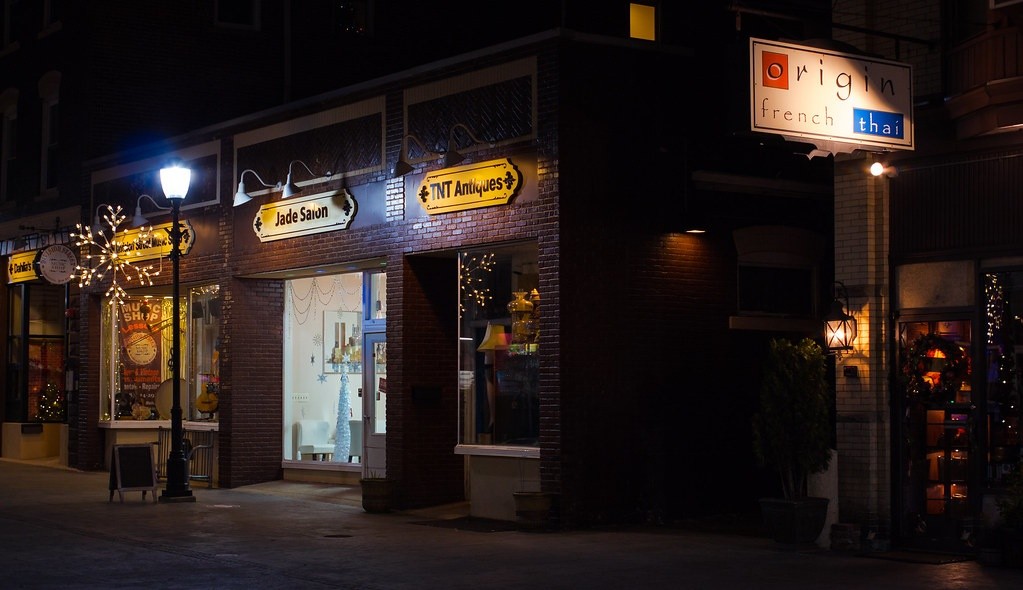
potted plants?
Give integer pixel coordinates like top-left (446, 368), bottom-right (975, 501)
top-left (751, 337), bottom-right (835, 546)
top-left (359, 471), bottom-right (394, 513)
top-left (995, 461), bottom-right (1023, 571)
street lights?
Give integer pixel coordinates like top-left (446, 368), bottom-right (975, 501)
top-left (158, 158), bottom-right (192, 498)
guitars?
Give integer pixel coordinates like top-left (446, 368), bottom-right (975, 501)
top-left (194, 351), bottom-right (219, 412)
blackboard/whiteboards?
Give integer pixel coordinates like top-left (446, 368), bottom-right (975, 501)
top-left (113, 443), bottom-right (158, 492)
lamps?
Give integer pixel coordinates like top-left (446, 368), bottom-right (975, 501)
top-left (13, 223), bottom-right (47, 251)
top-left (131, 195), bottom-right (174, 229)
top-left (232, 169), bottom-right (280, 208)
top-left (281, 160), bottom-right (316, 199)
top-left (440, 123), bottom-right (486, 170)
top-left (393, 134), bottom-right (433, 180)
top-left (476, 321), bottom-right (511, 448)
top-left (818, 280), bottom-right (857, 356)
top-left (89, 204), bottom-right (124, 236)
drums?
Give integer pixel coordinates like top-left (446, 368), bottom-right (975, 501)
top-left (155, 377), bottom-right (187, 419)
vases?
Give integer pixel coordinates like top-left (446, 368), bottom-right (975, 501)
top-left (511, 489), bottom-right (552, 529)
